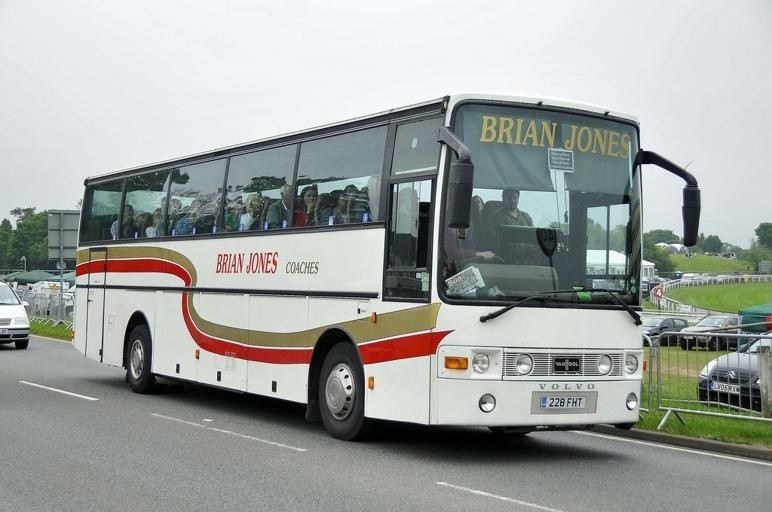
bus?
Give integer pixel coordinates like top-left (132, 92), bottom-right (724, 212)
top-left (658, 271), bottom-right (682, 279)
top-left (75, 96), bottom-right (698, 441)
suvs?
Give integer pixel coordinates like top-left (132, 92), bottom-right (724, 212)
top-left (678, 314), bottom-right (739, 351)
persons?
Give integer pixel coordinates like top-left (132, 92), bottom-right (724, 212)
top-left (469, 187), bottom-right (538, 264)
top-left (470, 194), bottom-right (485, 224)
top-left (441, 224), bottom-right (497, 275)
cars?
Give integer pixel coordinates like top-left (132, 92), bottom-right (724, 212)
top-left (695, 331), bottom-right (772, 412)
top-left (0, 282), bottom-right (29, 350)
top-left (681, 273), bottom-right (743, 285)
top-left (642, 316), bottom-right (688, 347)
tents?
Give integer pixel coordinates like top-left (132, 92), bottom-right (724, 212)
top-left (738, 302), bottom-right (772, 334)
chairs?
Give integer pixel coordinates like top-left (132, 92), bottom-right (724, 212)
top-left (479, 201), bottom-right (503, 258)
top-left (110, 192), bottom-right (375, 240)
top-left (389, 190), bottom-right (420, 268)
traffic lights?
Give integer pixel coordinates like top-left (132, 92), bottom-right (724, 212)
top-left (19, 257), bottom-right (26, 272)
